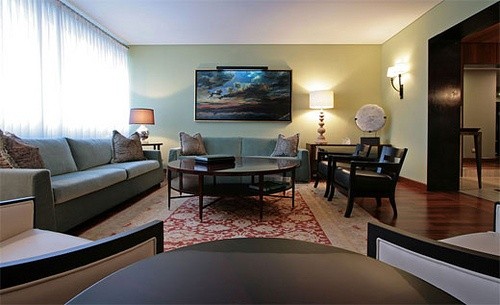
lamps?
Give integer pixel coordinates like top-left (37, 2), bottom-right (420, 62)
top-left (128, 108), bottom-right (155, 144)
top-left (308, 89), bottom-right (335, 143)
top-left (386, 64), bottom-right (404, 99)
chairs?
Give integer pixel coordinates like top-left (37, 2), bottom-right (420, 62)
top-left (0, 194), bottom-right (165, 305)
top-left (326, 145), bottom-right (409, 220)
top-left (367, 201), bottom-right (500, 305)
top-left (314, 143), bottom-right (373, 198)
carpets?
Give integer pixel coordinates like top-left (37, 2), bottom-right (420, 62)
top-left (76, 180), bottom-right (383, 257)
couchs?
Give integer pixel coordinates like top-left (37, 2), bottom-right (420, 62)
top-left (167, 135), bottom-right (310, 184)
top-left (0, 137), bottom-right (164, 232)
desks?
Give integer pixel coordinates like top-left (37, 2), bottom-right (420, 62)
top-left (460, 127), bottom-right (483, 190)
top-left (63, 237), bottom-right (467, 305)
top-left (360, 137), bottom-right (380, 146)
top-left (305, 142), bottom-right (328, 183)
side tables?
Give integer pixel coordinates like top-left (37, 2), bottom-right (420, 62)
top-left (141, 143), bottom-right (163, 150)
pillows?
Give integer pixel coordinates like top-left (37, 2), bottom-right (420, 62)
top-left (179, 131), bottom-right (209, 157)
top-left (0, 129), bottom-right (47, 169)
top-left (271, 134), bottom-right (300, 158)
top-left (109, 129), bottom-right (148, 164)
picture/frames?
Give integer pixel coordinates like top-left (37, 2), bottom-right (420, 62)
top-left (193, 69), bottom-right (293, 122)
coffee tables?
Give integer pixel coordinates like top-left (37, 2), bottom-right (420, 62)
top-left (166, 156), bottom-right (301, 224)
